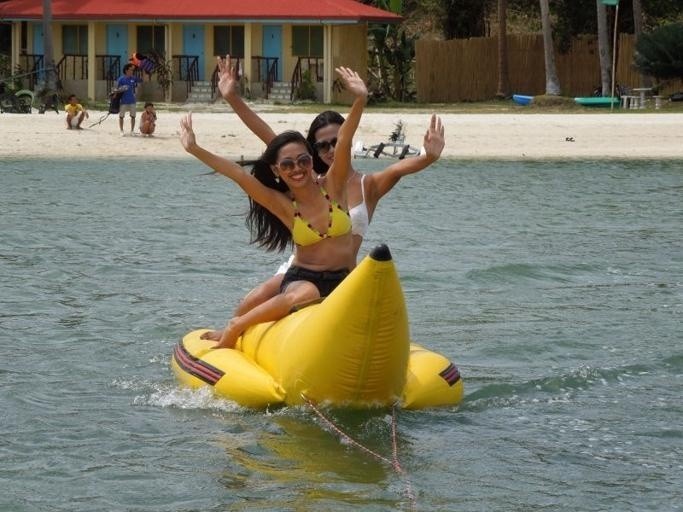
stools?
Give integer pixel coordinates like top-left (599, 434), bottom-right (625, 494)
top-left (618, 87), bottom-right (669, 111)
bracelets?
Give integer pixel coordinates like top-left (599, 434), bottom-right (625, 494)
top-left (153, 113), bottom-right (156, 117)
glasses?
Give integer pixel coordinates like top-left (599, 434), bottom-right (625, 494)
top-left (313, 138), bottom-right (338, 154)
top-left (273, 154), bottom-right (315, 173)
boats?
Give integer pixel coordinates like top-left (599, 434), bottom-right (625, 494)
top-left (512, 94), bottom-right (619, 109)
top-left (169, 244), bottom-right (466, 410)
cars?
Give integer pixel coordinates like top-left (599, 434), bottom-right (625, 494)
top-left (366, 27), bottom-right (391, 46)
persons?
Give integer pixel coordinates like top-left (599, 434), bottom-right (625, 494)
top-left (65, 94), bottom-right (89, 129)
top-left (114, 64), bottom-right (145, 137)
top-left (176, 66), bottom-right (368, 350)
top-left (200, 54), bottom-right (445, 341)
top-left (139, 103), bottom-right (157, 137)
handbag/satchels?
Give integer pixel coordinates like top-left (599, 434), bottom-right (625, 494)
top-left (108, 89), bottom-right (122, 115)
